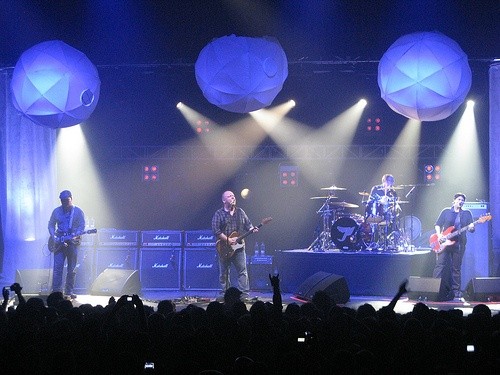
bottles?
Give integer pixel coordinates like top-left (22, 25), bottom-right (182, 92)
top-left (260, 241), bottom-right (265, 254)
top-left (255, 242), bottom-right (260, 254)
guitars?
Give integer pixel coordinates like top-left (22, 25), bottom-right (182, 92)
top-left (428, 212), bottom-right (493, 255)
top-left (47, 228), bottom-right (97, 253)
top-left (214, 215), bottom-right (272, 260)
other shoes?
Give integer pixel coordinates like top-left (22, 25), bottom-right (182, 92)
top-left (68, 293), bottom-right (77, 299)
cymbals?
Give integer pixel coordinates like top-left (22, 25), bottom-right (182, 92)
top-left (391, 187), bottom-right (404, 190)
top-left (322, 201), bottom-right (360, 208)
top-left (358, 192), bottom-right (370, 196)
top-left (309, 196), bottom-right (339, 200)
top-left (377, 187), bottom-right (393, 190)
top-left (391, 201), bottom-right (409, 204)
top-left (320, 186), bottom-right (348, 192)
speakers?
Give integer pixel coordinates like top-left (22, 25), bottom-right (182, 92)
top-left (407, 275), bottom-right (441, 301)
top-left (90, 268), bottom-right (140, 297)
top-left (14, 267), bottom-right (53, 295)
top-left (463, 277), bottom-right (500, 303)
top-left (96, 247), bottom-right (137, 275)
top-left (183, 248), bottom-right (224, 291)
top-left (139, 248), bottom-right (182, 291)
top-left (294, 271), bottom-right (350, 304)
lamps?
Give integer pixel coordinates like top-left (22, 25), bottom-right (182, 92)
top-left (423, 162), bottom-right (442, 184)
top-left (281, 167), bottom-right (300, 188)
top-left (142, 163), bottom-right (161, 185)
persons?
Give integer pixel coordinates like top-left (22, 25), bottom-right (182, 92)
top-left (212, 190), bottom-right (259, 302)
top-left (366, 174), bottom-right (399, 232)
top-left (435, 193), bottom-right (475, 298)
top-left (48, 190), bottom-right (86, 297)
top-left (0, 273), bottom-right (500, 375)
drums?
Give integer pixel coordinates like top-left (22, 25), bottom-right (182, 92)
top-left (371, 201), bottom-right (389, 216)
top-left (378, 213), bottom-right (394, 226)
top-left (330, 214), bottom-right (372, 252)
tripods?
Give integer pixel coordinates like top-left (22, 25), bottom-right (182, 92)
top-left (306, 200), bottom-right (338, 251)
top-left (365, 188), bottom-right (411, 253)
